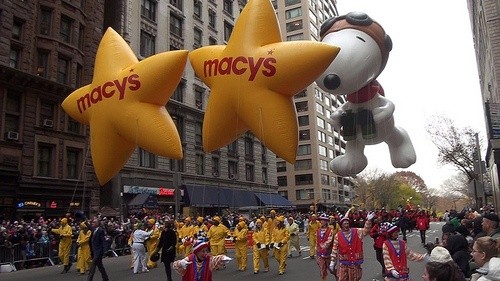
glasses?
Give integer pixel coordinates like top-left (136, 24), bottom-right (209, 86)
top-left (472, 249), bottom-right (481, 254)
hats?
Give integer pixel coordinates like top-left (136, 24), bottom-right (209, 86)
top-left (81, 222), bottom-right (87, 228)
top-left (333, 205), bottom-right (356, 224)
top-left (385, 222), bottom-right (399, 234)
top-left (318, 213), bottom-right (330, 220)
top-left (61, 218), bottom-right (67, 224)
top-left (312, 214), bottom-right (316, 219)
top-left (149, 219), bottom-right (155, 224)
top-left (197, 216), bottom-right (204, 224)
top-left (214, 217), bottom-right (221, 222)
top-left (137, 223), bottom-right (144, 228)
top-left (185, 217), bottom-right (191, 222)
top-left (193, 239), bottom-right (209, 254)
top-left (238, 209), bottom-right (285, 229)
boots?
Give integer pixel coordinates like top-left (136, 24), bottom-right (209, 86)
top-left (62, 265), bottom-right (68, 273)
top-left (69, 262), bottom-right (72, 269)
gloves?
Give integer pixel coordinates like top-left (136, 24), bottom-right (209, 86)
top-left (225, 232), bottom-right (296, 249)
top-left (367, 212), bottom-right (375, 219)
top-left (391, 270), bottom-right (400, 279)
top-left (329, 261), bottom-right (335, 271)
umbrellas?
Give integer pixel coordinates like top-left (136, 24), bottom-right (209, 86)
top-left (189, 0), bottom-right (341, 165)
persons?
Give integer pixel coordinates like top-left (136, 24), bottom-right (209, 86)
top-left (173, 233), bottom-right (232, 281)
top-left (0, 199), bottom-right (500, 281)
top-left (383, 222), bottom-right (428, 281)
top-left (329, 210), bottom-right (375, 281)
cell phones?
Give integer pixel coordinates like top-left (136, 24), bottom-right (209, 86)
top-left (435, 237), bottom-right (438, 243)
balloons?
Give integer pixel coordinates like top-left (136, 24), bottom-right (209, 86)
top-left (61, 26), bottom-right (189, 185)
top-left (315, 11), bottom-right (416, 176)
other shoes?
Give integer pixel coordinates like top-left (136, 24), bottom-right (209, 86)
top-left (237, 266), bottom-right (283, 275)
top-left (299, 252), bottom-right (302, 255)
top-left (310, 256), bottom-right (313, 259)
top-left (145, 270), bottom-right (149, 273)
top-left (288, 255), bottom-right (292, 258)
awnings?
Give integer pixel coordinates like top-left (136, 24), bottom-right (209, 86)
top-left (184, 186), bottom-right (295, 208)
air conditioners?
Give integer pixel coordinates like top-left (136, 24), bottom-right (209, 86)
top-left (43, 119), bottom-right (54, 127)
top-left (8, 131), bottom-right (19, 140)
